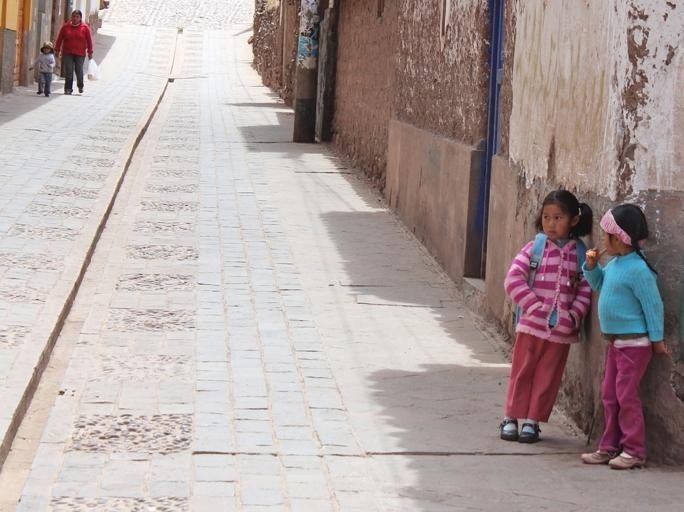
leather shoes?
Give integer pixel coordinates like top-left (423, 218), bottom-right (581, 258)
top-left (520, 423), bottom-right (541, 443)
top-left (501, 419), bottom-right (518, 441)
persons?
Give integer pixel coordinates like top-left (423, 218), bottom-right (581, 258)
top-left (29, 41), bottom-right (57, 97)
top-left (579, 202), bottom-right (673, 470)
top-left (54, 9), bottom-right (94, 95)
top-left (498, 190), bottom-right (592, 444)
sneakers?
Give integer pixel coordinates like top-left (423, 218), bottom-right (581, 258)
top-left (580, 452), bottom-right (646, 469)
top-left (64, 87), bottom-right (83, 95)
top-left (36, 92), bottom-right (49, 96)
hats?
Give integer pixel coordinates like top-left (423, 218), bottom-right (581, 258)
top-left (41, 41), bottom-right (53, 53)
